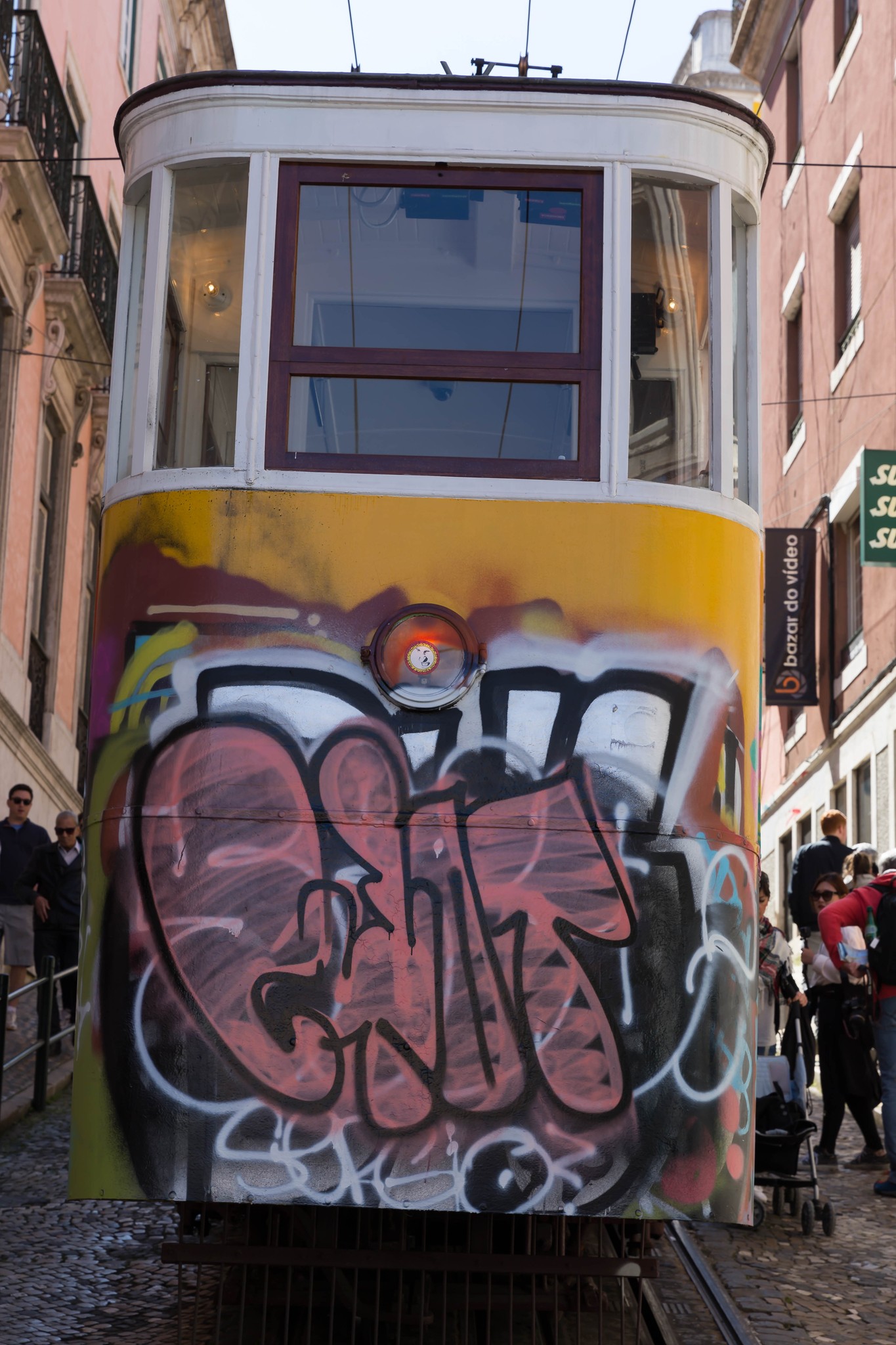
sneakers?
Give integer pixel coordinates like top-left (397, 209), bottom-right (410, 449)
top-left (844, 1146), bottom-right (891, 1171)
top-left (796, 1145), bottom-right (839, 1172)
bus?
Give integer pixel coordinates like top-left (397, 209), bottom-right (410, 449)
top-left (62, 56), bottom-right (763, 1239)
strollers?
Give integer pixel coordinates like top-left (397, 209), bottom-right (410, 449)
top-left (747, 972), bottom-right (836, 1236)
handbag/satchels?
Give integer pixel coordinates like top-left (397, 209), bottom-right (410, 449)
top-left (841, 996), bottom-right (869, 1040)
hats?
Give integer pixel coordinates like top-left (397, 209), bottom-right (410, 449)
top-left (851, 842), bottom-right (878, 863)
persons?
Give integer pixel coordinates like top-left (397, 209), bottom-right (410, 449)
top-left (23, 810), bottom-right (83, 1055)
top-left (755, 810), bottom-right (896, 1199)
top-left (0, 783), bottom-right (52, 1028)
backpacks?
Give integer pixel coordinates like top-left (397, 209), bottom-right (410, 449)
top-left (865, 881), bottom-right (896, 987)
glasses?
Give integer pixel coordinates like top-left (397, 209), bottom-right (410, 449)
top-left (807, 888), bottom-right (840, 901)
top-left (54, 825), bottom-right (78, 835)
top-left (12, 796), bottom-right (32, 806)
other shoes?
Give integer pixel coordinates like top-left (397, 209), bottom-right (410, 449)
top-left (873, 1179), bottom-right (896, 1198)
top-left (6, 1009), bottom-right (18, 1030)
